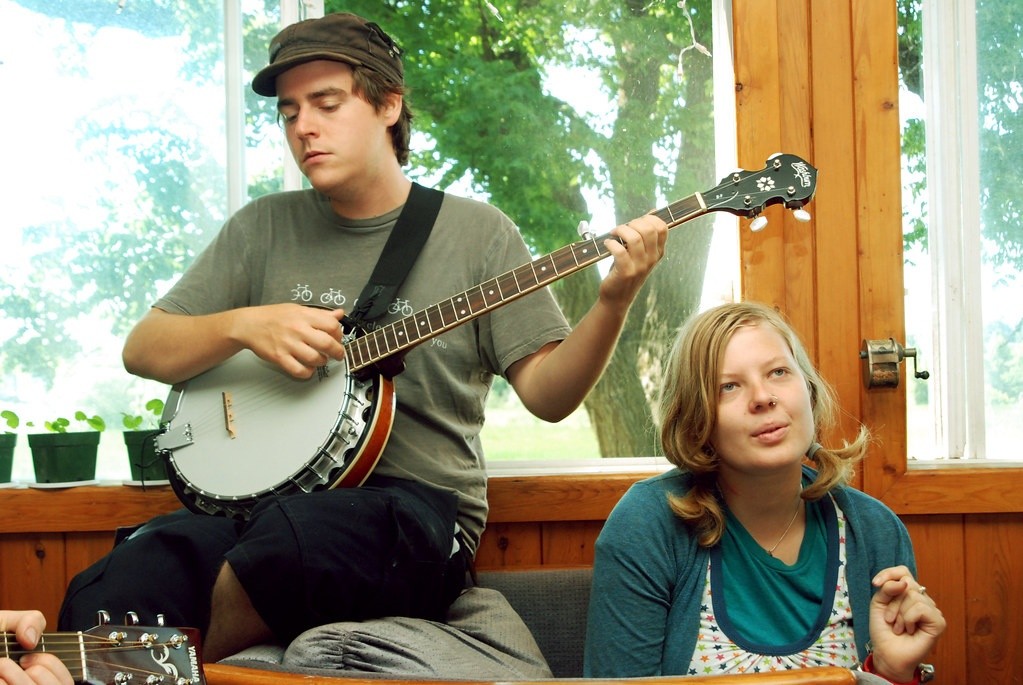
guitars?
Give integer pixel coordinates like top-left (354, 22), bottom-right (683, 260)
top-left (0, 609), bottom-right (209, 685)
top-left (151, 152), bottom-right (820, 523)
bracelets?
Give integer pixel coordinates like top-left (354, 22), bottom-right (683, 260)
top-left (918, 662), bottom-right (934, 682)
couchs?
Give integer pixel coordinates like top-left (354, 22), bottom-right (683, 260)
top-left (216, 568), bottom-right (595, 680)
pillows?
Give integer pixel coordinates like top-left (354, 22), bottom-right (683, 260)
top-left (280, 585), bottom-right (555, 680)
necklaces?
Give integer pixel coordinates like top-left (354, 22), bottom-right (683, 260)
top-left (767, 485), bottom-right (804, 556)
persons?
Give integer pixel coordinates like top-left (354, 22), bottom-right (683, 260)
top-left (57, 13), bottom-right (669, 662)
top-left (0, 610), bottom-right (74, 685)
top-left (585, 302), bottom-right (946, 685)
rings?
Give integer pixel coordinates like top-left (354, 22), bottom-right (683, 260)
top-left (917, 586), bottom-right (927, 593)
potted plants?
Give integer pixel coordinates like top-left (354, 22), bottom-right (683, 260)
top-left (0, 409), bottom-right (20, 483)
top-left (25, 411), bottom-right (105, 483)
top-left (119, 397), bottom-right (168, 481)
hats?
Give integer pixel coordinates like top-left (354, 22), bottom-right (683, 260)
top-left (251, 13), bottom-right (403, 92)
